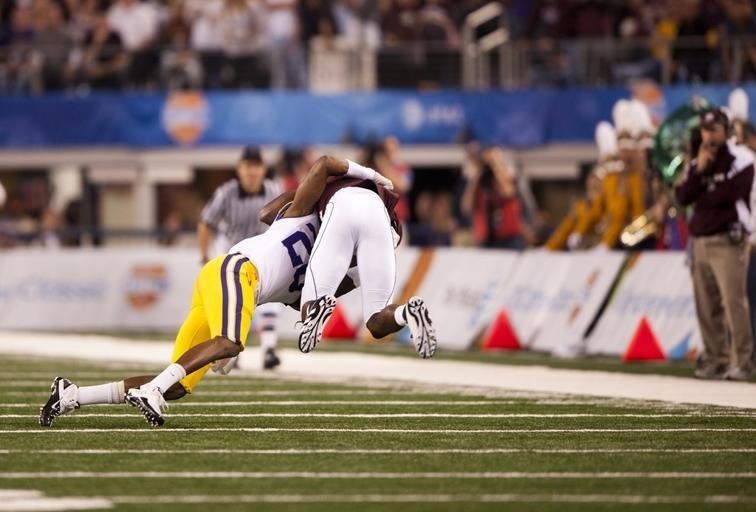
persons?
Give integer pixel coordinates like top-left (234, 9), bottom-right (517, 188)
top-left (23, 80), bottom-right (756, 255)
top-left (1, 1), bottom-right (755, 95)
top-left (258, 173), bottom-right (440, 360)
top-left (196, 144), bottom-right (284, 372)
top-left (668, 106), bottom-right (756, 384)
top-left (37, 155), bottom-right (404, 430)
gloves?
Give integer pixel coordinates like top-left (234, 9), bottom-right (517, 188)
top-left (373, 171), bottom-right (394, 192)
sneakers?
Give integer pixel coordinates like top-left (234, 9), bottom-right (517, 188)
top-left (407, 296), bottom-right (437, 358)
top-left (39, 376), bottom-right (80, 427)
top-left (694, 361), bottom-right (756, 379)
top-left (298, 294), bottom-right (337, 353)
top-left (124, 385), bottom-right (165, 427)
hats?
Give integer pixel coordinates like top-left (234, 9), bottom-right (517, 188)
top-left (698, 108), bottom-right (729, 127)
